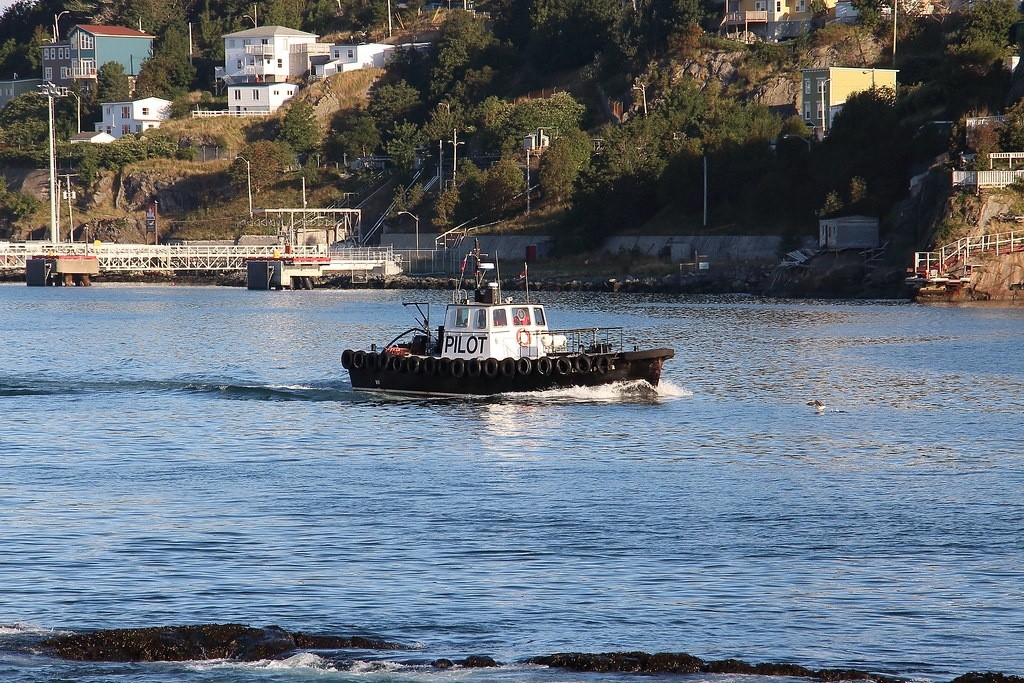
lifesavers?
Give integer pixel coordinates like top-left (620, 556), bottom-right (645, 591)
top-left (422, 356), bottom-right (437, 377)
top-left (466, 358), bottom-right (482, 379)
top-left (406, 355), bottom-right (422, 375)
top-left (341, 349), bottom-right (355, 369)
top-left (554, 357), bottom-right (572, 377)
top-left (392, 355), bottom-right (407, 373)
top-left (482, 357), bottom-right (499, 378)
top-left (449, 358), bottom-right (466, 379)
top-left (365, 352), bottom-right (381, 372)
top-left (536, 356), bottom-right (553, 376)
top-left (517, 357), bottom-right (532, 375)
top-left (378, 352), bottom-right (393, 372)
top-left (499, 357), bottom-right (516, 377)
top-left (353, 350), bottom-right (368, 370)
top-left (436, 357), bottom-right (451, 376)
top-left (575, 354), bottom-right (593, 375)
top-left (516, 328), bottom-right (532, 347)
top-left (596, 355), bottom-right (612, 375)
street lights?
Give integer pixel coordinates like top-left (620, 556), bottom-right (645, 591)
top-left (398, 211), bottom-right (419, 258)
top-left (64, 90), bottom-right (81, 134)
top-left (234, 156), bottom-right (253, 219)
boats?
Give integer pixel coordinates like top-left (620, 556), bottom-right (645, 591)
top-left (339, 236), bottom-right (676, 399)
